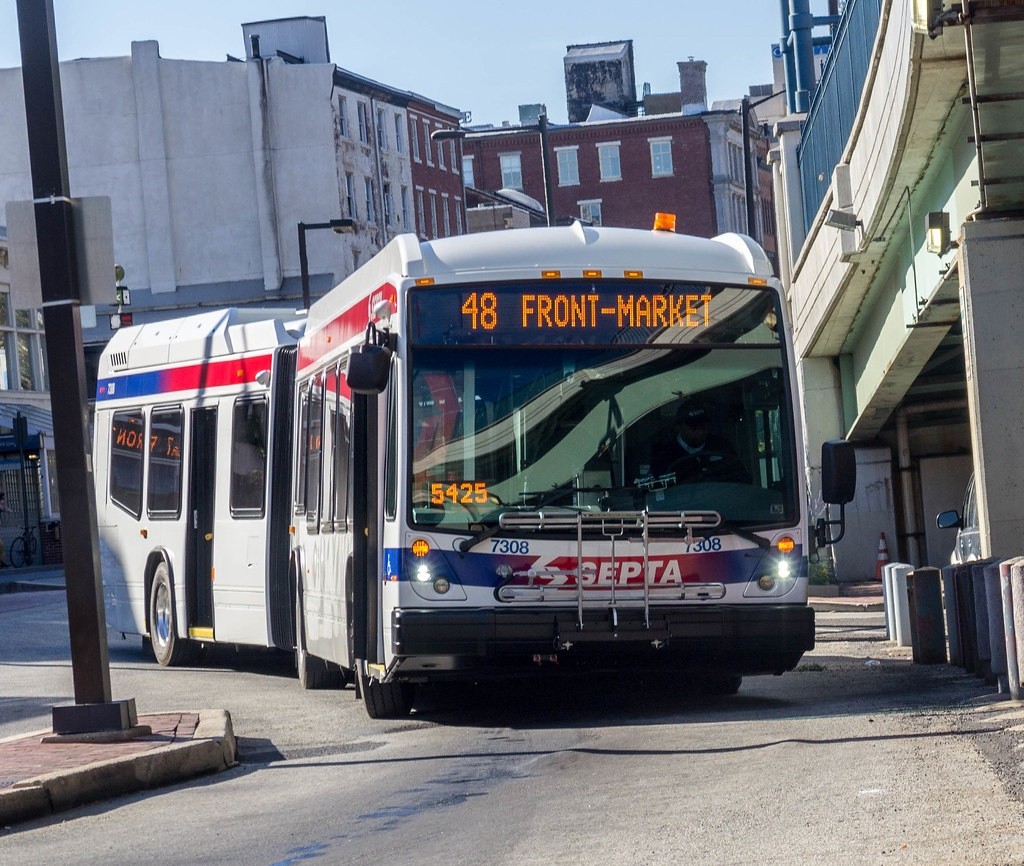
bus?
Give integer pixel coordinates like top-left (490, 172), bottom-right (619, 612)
top-left (86, 210), bottom-right (858, 722)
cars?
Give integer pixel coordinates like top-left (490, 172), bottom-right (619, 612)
top-left (935, 469), bottom-right (982, 568)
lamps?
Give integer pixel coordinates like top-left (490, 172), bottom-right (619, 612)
top-left (911, 0), bottom-right (967, 40)
top-left (924, 210), bottom-right (960, 259)
top-left (825, 210), bottom-right (863, 232)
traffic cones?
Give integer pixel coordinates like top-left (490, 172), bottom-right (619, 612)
top-left (869, 532), bottom-right (892, 582)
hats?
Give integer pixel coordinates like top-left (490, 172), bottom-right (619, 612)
top-left (676, 399), bottom-right (712, 426)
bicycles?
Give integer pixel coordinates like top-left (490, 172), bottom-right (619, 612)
top-left (10, 525), bottom-right (38, 569)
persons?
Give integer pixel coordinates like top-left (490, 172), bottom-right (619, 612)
top-left (647, 400), bottom-right (745, 482)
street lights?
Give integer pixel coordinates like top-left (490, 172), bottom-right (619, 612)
top-left (295, 218), bottom-right (359, 312)
top-left (430, 112), bottom-right (556, 227)
